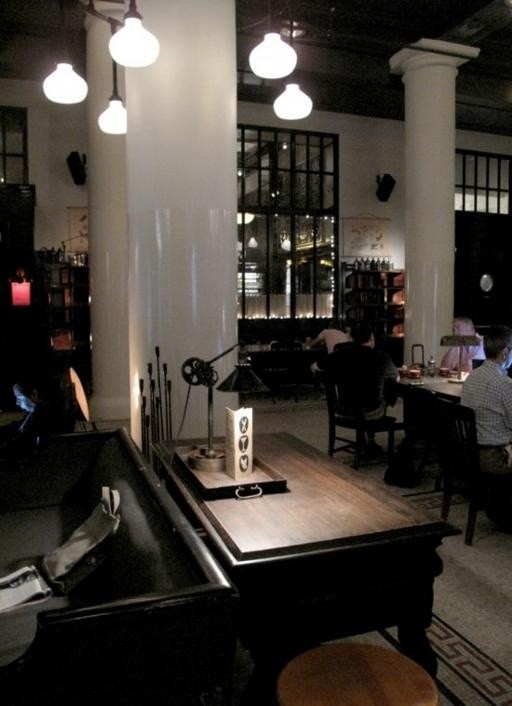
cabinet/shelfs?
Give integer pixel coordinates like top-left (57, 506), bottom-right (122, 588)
top-left (30, 262), bottom-right (89, 351)
top-left (341, 262), bottom-right (405, 334)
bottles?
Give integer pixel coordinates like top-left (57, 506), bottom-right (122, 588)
top-left (428, 356), bottom-right (436, 375)
top-left (71, 251), bottom-right (88, 266)
top-left (354, 256), bottom-right (394, 271)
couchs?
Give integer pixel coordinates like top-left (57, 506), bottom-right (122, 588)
top-left (0, 427), bottom-right (241, 704)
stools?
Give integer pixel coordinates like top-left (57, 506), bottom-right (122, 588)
top-left (277, 644), bottom-right (440, 706)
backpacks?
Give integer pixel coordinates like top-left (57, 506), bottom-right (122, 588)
top-left (383, 436), bottom-right (430, 488)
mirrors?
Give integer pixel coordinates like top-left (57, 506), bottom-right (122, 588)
top-left (479, 273), bottom-right (494, 293)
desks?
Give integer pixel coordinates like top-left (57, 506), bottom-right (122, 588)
top-left (147, 430), bottom-right (462, 686)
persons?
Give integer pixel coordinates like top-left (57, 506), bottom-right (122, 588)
top-left (1, 378), bottom-right (46, 443)
top-left (462, 322), bottom-right (511, 476)
top-left (310, 327), bottom-right (352, 380)
top-left (312, 325), bottom-right (405, 454)
top-left (442, 319), bottom-right (487, 376)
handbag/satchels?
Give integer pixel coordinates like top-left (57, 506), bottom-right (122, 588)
top-left (39, 502), bottom-right (121, 593)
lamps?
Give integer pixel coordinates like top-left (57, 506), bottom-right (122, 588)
top-left (6, 269), bottom-right (31, 308)
top-left (106, 0), bottom-right (160, 68)
top-left (96, 22), bottom-right (127, 135)
top-left (41, 0), bottom-right (89, 105)
top-left (29, 366), bottom-right (92, 433)
top-left (272, 0), bottom-right (314, 120)
top-left (249, 0), bottom-right (298, 80)
top-left (188, 334), bottom-right (271, 471)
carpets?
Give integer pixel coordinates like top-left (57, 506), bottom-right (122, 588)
top-left (375, 485), bottom-right (512, 706)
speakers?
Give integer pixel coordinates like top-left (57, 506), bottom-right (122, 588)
top-left (374, 172), bottom-right (397, 204)
top-left (65, 150), bottom-right (88, 187)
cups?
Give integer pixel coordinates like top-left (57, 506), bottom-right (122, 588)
top-left (397, 366), bottom-right (450, 379)
